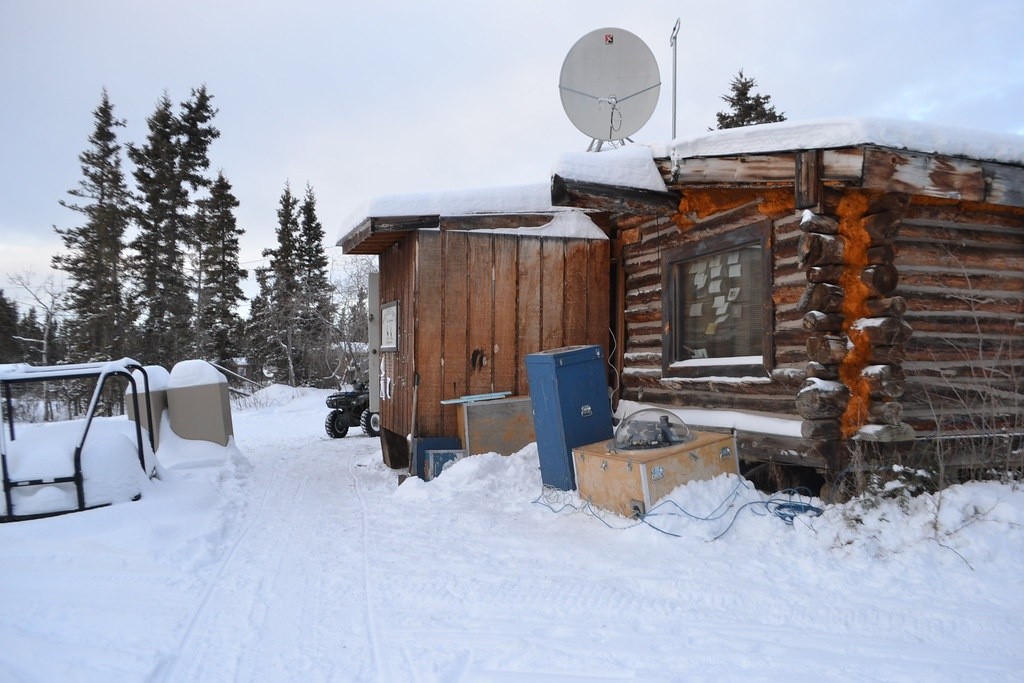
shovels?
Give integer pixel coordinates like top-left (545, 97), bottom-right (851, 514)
top-left (398, 373), bottom-right (419, 486)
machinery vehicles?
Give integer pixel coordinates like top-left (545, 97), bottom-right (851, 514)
top-left (324, 378), bottom-right (381, 438)
